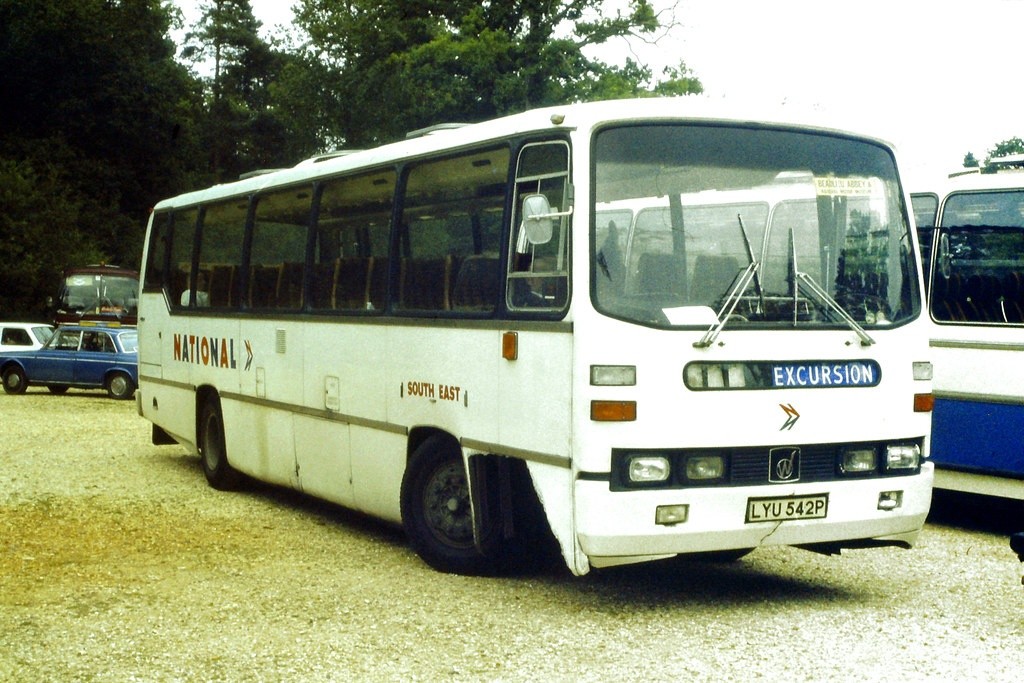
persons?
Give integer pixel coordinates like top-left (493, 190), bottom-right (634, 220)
top-left (521, 256), bottom-right (558, 306)
top-left (179, 270), bottom-right (210, 308)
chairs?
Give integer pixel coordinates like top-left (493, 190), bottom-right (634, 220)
top-left (843, 272), bottom-right (1022, 323)
top-left (164, 253), bottom-right (740, 324)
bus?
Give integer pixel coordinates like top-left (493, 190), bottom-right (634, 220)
top-left (135, 94), bottom-right (939, 579)
top-left (46, 261), bottom-right (141, 328)
top-left (558, 152), bottom-right (1024, 505)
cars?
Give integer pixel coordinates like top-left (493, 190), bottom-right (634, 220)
top-left (0, 321), bottom-right (81, 382)
top-left (0, 320), bottom-right (139, 400)
